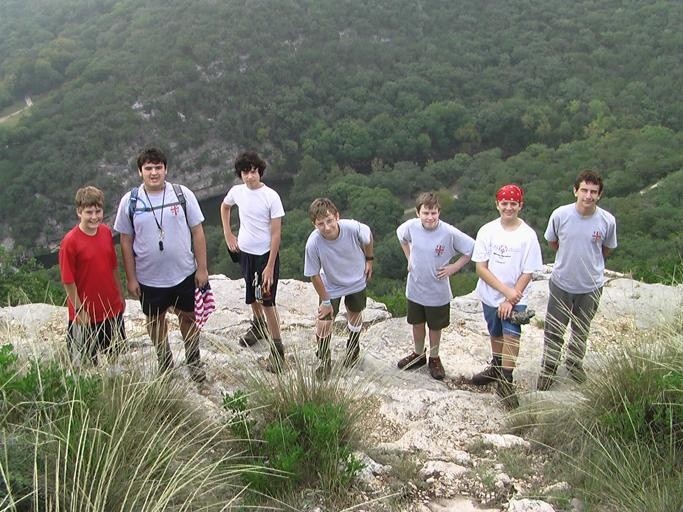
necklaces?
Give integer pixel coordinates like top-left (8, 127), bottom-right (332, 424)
top-left (143, 184), bottom-right (166, 251)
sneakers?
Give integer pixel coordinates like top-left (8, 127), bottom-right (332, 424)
top-left (565, 359), bottom-right (586, 384)
top-left (156, 344), bottom-right (174, 385)
top-left (428, 355), bottom-right (446, 380)
top-left (265, 340), bottom-right (286, 374)
top-left (185, 350), bottom-right (205, 382)
top-left (397, 349), bottom-right (426, 370)
top-left (537, 363), bottom-right (559, 391)
top-left (239, 316), bottom-right (268, 348)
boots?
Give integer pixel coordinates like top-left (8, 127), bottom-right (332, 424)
top-left (344, 328), bottom-right (360, 370)
top-left (315, 334), bottom-right (331, 381)
top-left (496, 365), bottom-right (520, 411)
top-left (472, 356), bottom-right (500, 386)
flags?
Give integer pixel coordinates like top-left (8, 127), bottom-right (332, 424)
top-left (194, 283), bottom-right (215, 330)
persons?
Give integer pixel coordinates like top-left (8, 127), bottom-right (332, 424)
top-left (220, 151), bottom-right (285, 374)
top-left (534, 170), bottom-right (618, 391)
top-left (470, 185), bottom-right (543, 410)
top-left (59, 186), bottom-right (128, 361)
top-left (304, 198), bottom-right (375, 379)
top-left (113, 146), bottom-right (209, 383)
top-left (396, 191), bottom-right (476, 380)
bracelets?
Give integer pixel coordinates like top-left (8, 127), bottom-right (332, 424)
top-left (75, 307), bottom-right (83, 313)
top-left (366, 256), bottom-right (374, 260)
